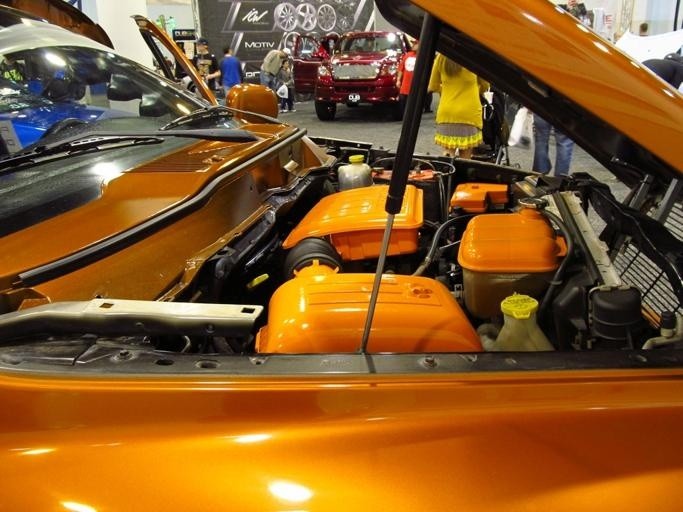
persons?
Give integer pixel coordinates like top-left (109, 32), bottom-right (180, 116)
top-left (217, 45), bottom-right (244, 98)
top-left (394, 40), bottom-right (419, 117)
top-left (191, 38), bottom-right (222, 90)
top-left (425, 51), bottom-right (492, 160)
top-left (638, 22), bottom-right (650, 37)
top-left (260, 46), bottom-right (292, 106)
top-left (557, 1), bottom-right (588, 20)
top-left (527, 110), bottom-right (576, 179)
top-left (277, 59), bottom-right (297, 114)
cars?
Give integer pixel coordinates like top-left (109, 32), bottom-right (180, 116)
top-left (292, 30), bottom-right (414, 121)
top-left (0, 0), bottom-right (681, 511)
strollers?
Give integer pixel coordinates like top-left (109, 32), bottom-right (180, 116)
top-left (455, 82), bottom-right (524, 166)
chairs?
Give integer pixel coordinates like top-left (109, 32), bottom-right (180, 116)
top-left (279, 109), bottom-right (296, 113)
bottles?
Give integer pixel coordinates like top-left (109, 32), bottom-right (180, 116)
top-left (410, 39), bottom-right (419, 44)
top-left (193, 38), bottom-right (208, 46)
top-left (283, 48), bottom-right (290, 55)
